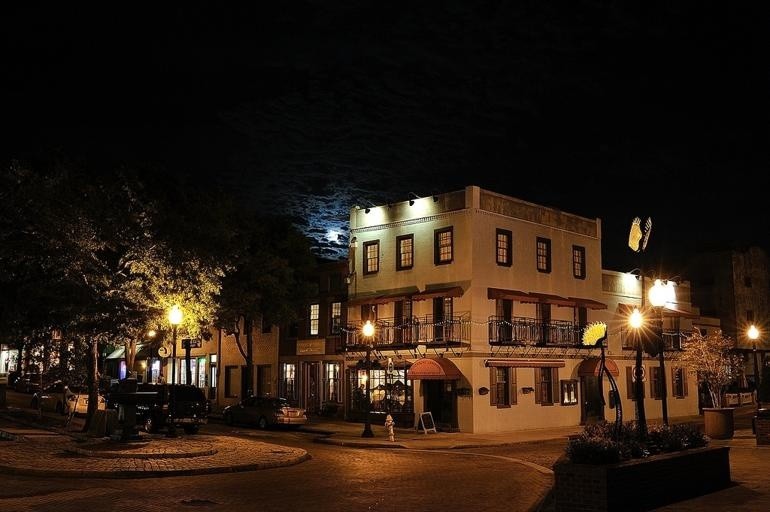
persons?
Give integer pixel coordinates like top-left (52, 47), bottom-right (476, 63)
top-left (159, 373), bottom-right (166, 384)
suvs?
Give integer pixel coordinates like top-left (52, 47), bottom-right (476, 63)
top-left (105, 381), bottom-right (208, 436)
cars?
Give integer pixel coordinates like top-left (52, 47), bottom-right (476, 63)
top-left (221, 396), bottom-right (308, 431)
top-left (0, 373), bottom-right (105, 415)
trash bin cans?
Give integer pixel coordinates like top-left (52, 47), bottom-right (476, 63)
top-left (753, 408), bottom-right (770, 447)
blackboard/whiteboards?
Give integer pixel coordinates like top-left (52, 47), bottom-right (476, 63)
top-left (420, 412), bottom-right (435, 431)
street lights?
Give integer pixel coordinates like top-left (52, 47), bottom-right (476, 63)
top-left (624, 267), bottom-right (677, 438)
top-left (361, 321), bottom-right (376, 436)
top-left (167, 305), bottom-right (181, 423)
top-left (747, 324), bottom-right (761, 407)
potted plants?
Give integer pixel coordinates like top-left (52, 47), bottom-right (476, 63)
top-left (670, 325), bottom-right (735, 440)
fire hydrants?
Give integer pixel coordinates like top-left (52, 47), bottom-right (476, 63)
top-left (386, 415), bottom-right (397, 442)
